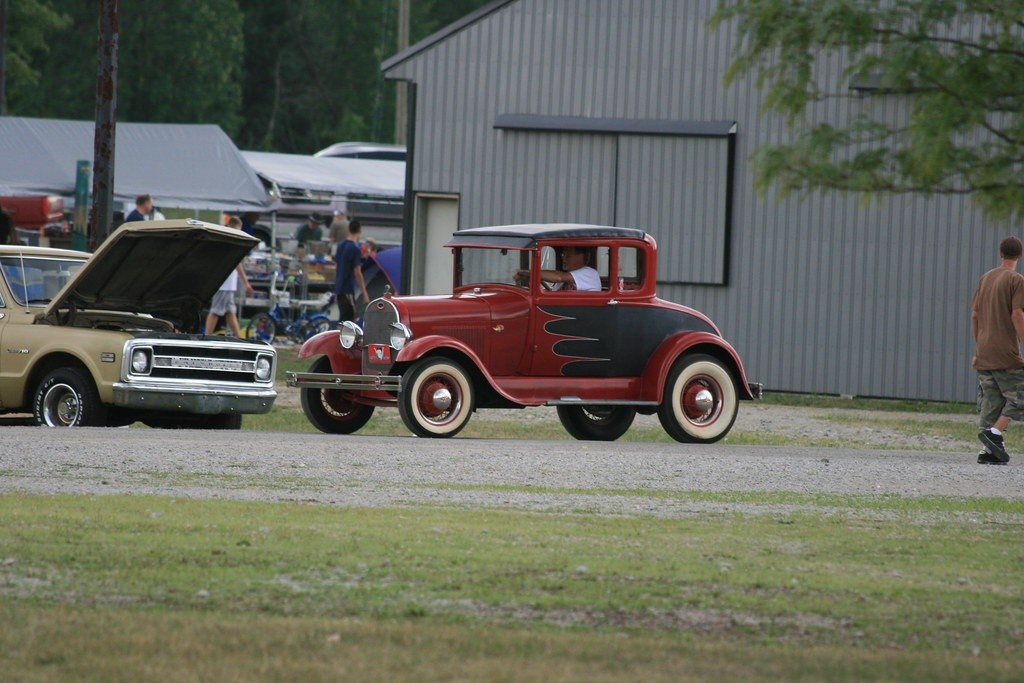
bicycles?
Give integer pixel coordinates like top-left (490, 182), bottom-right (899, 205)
top-left (246, 271), bottom-right (335, 345)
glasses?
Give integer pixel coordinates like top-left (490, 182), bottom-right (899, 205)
top-left (561, 252), bottom-right (582, 257)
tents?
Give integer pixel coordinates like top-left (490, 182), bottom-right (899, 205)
top-left (0, 116), bottom-right (281, 271)
top-left (238, 150), bottom-right (406, 271)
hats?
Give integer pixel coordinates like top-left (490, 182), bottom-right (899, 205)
top-left (308, 212), bottom-right (325, 224)
top-left (1002, 235), bottom-right (1023, 255)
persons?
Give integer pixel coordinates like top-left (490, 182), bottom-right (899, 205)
top-left (204, 216), bottom-right (253, 338)
top-left (335, 221), bottom-right (377, 322)
top-left (512, 246), bottom-right (601, 291)
top-left (125, 195), bottom-right (152, 221)
top-left (971, 236), bottom-right (1024, 464)
top-left (293, 210), bottom-right (350, 258)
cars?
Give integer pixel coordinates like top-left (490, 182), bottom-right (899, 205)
top-left (285, 223), bottom-right (764, 445)
top-left (0, 218), bottom-right (276, 430)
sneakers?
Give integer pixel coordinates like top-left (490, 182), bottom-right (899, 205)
top-left (977, 449), bottom-right (1008, 465)
top-left (978, 428), bottom-right (1010, 463)
